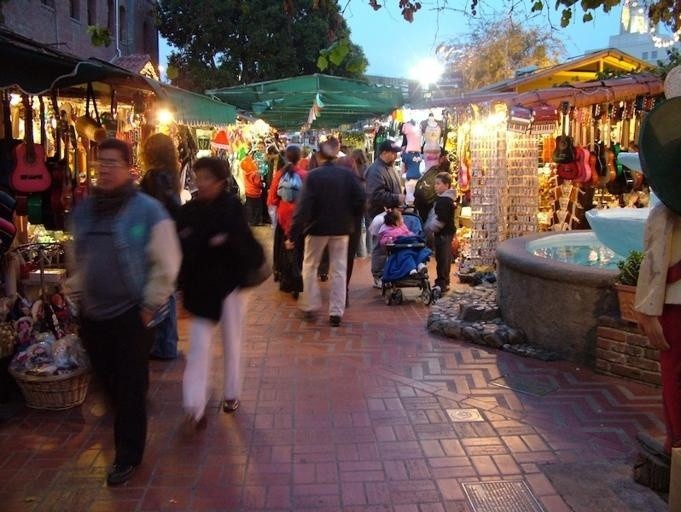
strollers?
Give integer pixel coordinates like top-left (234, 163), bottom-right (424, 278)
top-left (365, 206), bottom-right (442, 307)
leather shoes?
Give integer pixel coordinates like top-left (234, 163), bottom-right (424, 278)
top-left (107, 464), bottom-right (136, 484)
top-left (223, 399), bottom-right (240, 412)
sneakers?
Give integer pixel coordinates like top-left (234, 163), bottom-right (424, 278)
top-left (373, 262), bottom-right (450, 292)
top-left (330, 315), bottom-right (340, 327)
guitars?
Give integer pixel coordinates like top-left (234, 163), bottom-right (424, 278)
top-left (552, 93), bottom-right (656, 194)
top-left (0, 87), bottom-right (94, 230)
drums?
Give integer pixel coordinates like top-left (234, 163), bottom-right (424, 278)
top-left (75, 115), bottom-right (107, 145)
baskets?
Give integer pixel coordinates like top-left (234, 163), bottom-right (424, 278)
top-left (8, 363), bottom-right (91, 411)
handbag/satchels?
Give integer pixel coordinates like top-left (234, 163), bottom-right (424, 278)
top-left (277, 172), bottom-right (302, 202)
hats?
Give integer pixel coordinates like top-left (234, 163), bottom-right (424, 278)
top-left (379, 140), bottom-right (401, 152)
top-left (320, 137), bottom-right (342, 160)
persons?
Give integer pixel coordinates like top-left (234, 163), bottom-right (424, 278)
top-left (140, 133), bottom-right (181, 360)
top-left (634, 200), bottom-right (681, 457)
top-left (171, 158), bottom-right (244, 426)
top-left (272, 135), bottom-right (456, 327)
top-left (212, 129), bottom-right (271, 226)
top-left (68, 137), bottom-right (185, 487)
